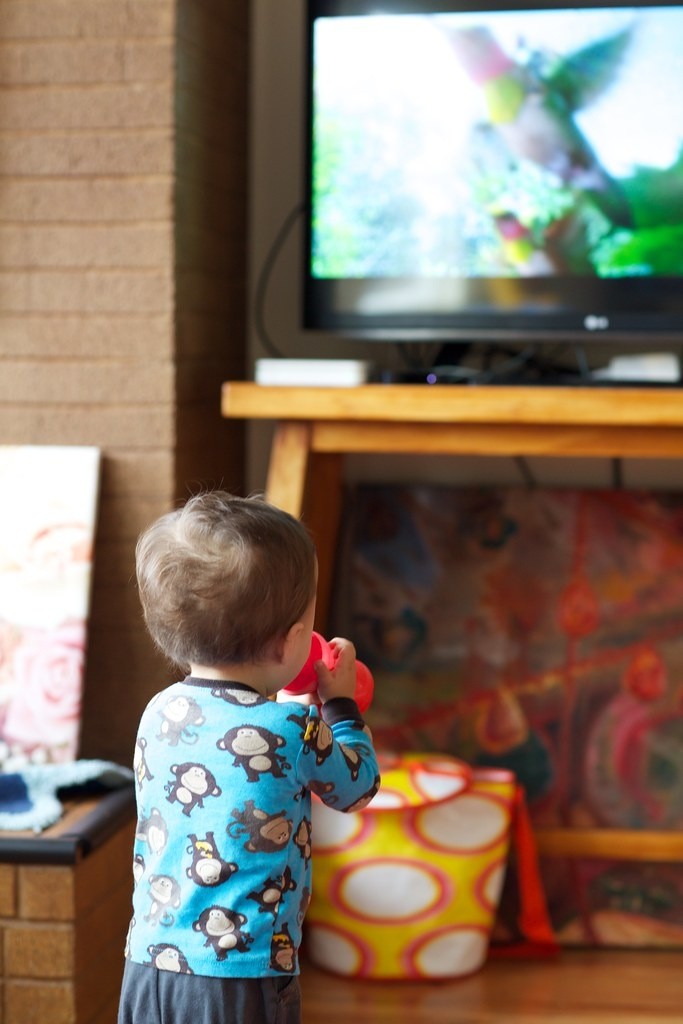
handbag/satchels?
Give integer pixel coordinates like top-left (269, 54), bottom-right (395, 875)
top-left (302, 752), bottom-right (517, 982)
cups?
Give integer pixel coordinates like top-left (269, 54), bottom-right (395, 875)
top-left (281, 630), bottom-right (374, 719)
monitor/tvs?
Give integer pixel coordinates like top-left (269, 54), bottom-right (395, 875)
top-left (301, 0), bottom-right (683, 390)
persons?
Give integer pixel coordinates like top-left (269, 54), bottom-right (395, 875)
top-left (117, 490), bottom-right (381, 1024)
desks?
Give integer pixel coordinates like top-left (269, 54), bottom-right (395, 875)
top-left (221, 379), bottom-right (683, 952)
